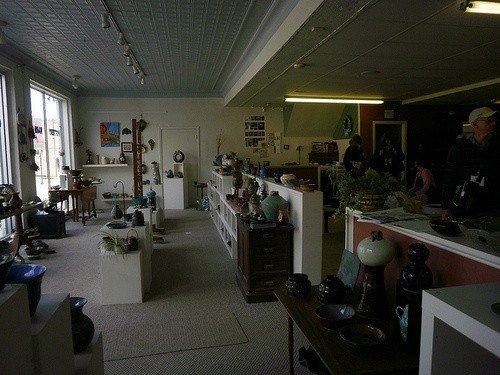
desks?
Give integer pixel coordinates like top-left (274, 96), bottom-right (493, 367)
top-left (49, 189), bottom-right (97, 226)
top-left (235, 214), bottom-right (295, 304)
top-left (163, 176), bottom-right (189, 210)
top-left (99, 220), bottom-right (151, 293)
top-left (0, 202), bottom-right (40, 267)
top-left (126, 205), bottom-right (152, 249)
top-left (419, 281), bottom-right (500, 375)
top-left (98, 247), bottom-right (145, 306)
top-left (191, 183), bottom-right (208, 210)
top-left (273, 285), bottom-right (418, 375)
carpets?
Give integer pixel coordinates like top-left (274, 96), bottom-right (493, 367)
top-left (100, 308), bottom-right (251, 362)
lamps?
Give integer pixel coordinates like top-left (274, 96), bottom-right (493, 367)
top-left (117, 33), bottom-right (125, 46)
top-left (123, 44), bottom-right (130, 57)
top-left (460, 0), bottom-right (500, 15)
top-left (72, 75), bottom-right (79, 89)
top-left (131, 65), bottom-right (139, 75)
top-left (126, 56), bottom-right (132, 66)
top-left (100, 12), bottom-right (111, 29)
top-left (283, 95), bottom-right (384, 105)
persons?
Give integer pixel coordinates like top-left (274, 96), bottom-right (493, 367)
top-left (430, 107), bottom-right (500, 220)
top-left (343, 135), bottom-right (366, 177)
top-left (371, 133), bottom-right (405, 183)
top-left (411, 160), bottom-right (437, 203)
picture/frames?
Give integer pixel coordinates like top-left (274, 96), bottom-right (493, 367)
top-left (121, 142), bottom-right (133, 153)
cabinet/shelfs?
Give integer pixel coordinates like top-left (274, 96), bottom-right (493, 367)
top-left (205, 169), bottom-right (237, 260)
top-left (0, 282), bottom-right (104, 375)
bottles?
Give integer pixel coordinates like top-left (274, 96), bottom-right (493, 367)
top-left (232, 172), bottom-right (289, 225)
top-left (243, 158), bottom-right (271, 177)
top-left (132, 211), bottom-right (144, 226)
top-left (119, 154), bottom-right (126, 164)
top-left (273, 171), bottom-right (296, 187)
top-left (110, 204), bottom-right (123, 219)
top-left (317, 275), bottom-right (346, 302)
top-left (70, 297), bottom-right (94, 354)
top-left (285, 274), bottom-right (311, 298)
top-left (0, 184), bottom-right (23, 215)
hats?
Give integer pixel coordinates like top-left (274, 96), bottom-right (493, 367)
top-left (469, 107), bottom-right (498, 123)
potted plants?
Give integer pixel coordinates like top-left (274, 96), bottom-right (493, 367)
top-left (93, 228), bottom-right (128, 261)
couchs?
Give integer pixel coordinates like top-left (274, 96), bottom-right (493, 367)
top-left (28, 208), bottom-right (65, 238)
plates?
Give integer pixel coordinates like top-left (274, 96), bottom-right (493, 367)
top-left (106, 214), bottom-right (133, 228)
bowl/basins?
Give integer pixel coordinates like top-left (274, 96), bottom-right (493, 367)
top-left (420, 202), bottom-right (442, 216)
top-left (285, 178), bottom-right (316, 192)
top-left (343, 324), bottom-right (386, 350)
top-left (132, 196), bottom-right (148, 205)
top-left (103, 193), bottom-right (110, 199)
top-left (430, 219), bottom-right (462, 237)
top-left (313, 303), bottom-right (355, 330)
top-left (62, 169), bottom-right (93, 188)
